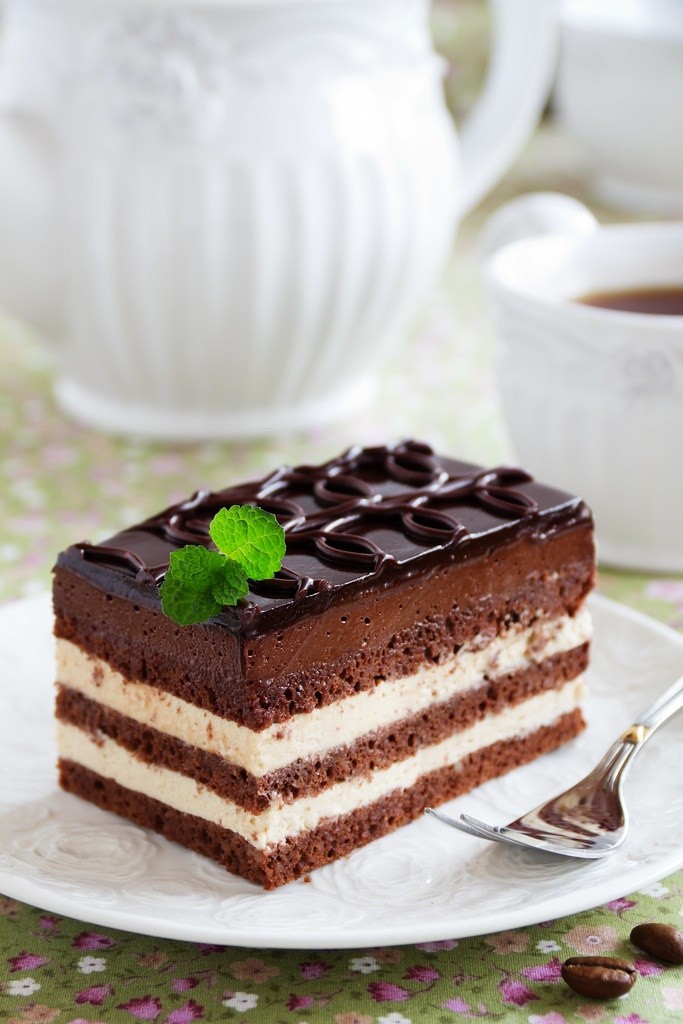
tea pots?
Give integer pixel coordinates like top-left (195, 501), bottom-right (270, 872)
top-left (0, 0), bottom-right (560, 443)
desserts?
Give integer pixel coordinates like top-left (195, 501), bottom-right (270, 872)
top-left (50, 439), bottom-right (594, 891)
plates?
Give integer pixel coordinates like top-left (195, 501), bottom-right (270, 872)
top-left (0, 589), bottom-right (683, 949)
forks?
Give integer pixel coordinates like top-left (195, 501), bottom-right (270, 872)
top-left (424, 678), bottom-right (683, 859)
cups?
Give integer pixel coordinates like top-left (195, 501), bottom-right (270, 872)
top-left (551, 0), bottom-right (682, 214)
top-left (475, 192), bottom-right (683, 580)
